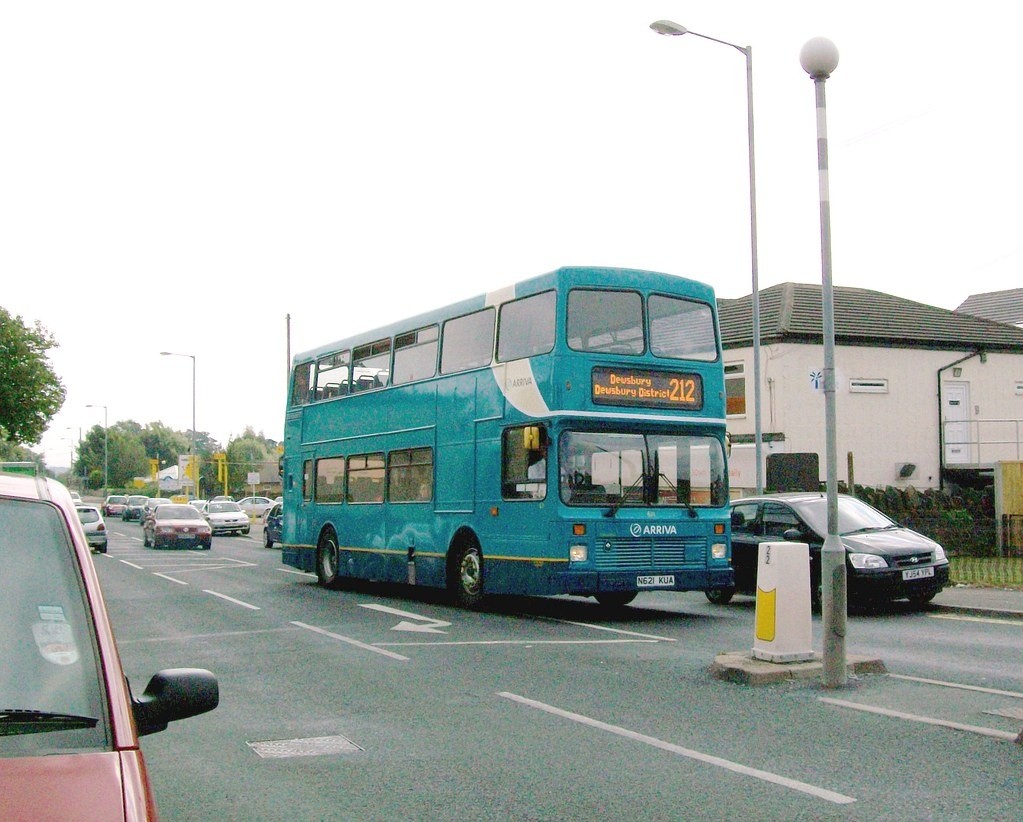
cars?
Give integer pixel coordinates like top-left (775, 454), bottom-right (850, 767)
top-left (121, 495), bottom-right (151, 522)
top-left (102, 495), bottom-right (128, 517)
top-left (187, 499), bottom-right (209, 514)
top-left (201, 501), bottom-right (251, 537)
top-left (706, 491), bottom-right (950, 617)
top-left (238, 497), bottom-right (280, 519)
top-left (0, 460), bottom-right (221, 821)
top-left (74, 506), bottom-right (110, 554)
top-left (212, 495), bottom-right (238, 506)
top-left (261, 501), bottom-right (283, 549)
top-left (142, 502), bottom-right (213, 551)
top-left (138, 497), bottom-right (173, 527)
top-left (69, 491), bottom-right (82, 507)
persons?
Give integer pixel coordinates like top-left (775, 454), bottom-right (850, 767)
top-left (415, 480), bottom-right (432, 501)
top-left (528, 437), bottom-right (571, 503)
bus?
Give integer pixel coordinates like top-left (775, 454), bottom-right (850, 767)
top-left (280, 265), bottom-right (738, 614)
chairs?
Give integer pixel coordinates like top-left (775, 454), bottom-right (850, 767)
top-left (609, 343), bottom-right (662, 358)
top-left (310, 370), bottom-right (389, 403)
top-left (309, 475), bottom-right (374, 503)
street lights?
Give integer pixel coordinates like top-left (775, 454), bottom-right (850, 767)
top-left (798, 37), bottom-right (848, 693)
top-left (160, 351), bottom-right (197, 501)
top-left (67, 427), bottom-right (82, 448)
top-left (62, 438), bottom-right (74, 470)
top-left (84, 404), bottom-right (108, 499)
top-left (649, 19), bottom-right (763, 495)
